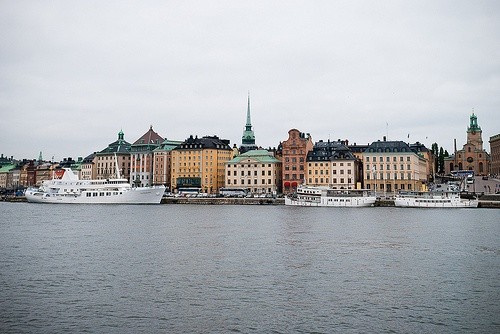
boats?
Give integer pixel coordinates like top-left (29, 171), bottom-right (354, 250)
top-left (392, 188), bottom-right (479, 210)
top-left (283, 186), bottom-right (377, 208)
top-left (479, 193), bottom-right (500, 208)
top-left (24, 153), bottom-right (167, 205)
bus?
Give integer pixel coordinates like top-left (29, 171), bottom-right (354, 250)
top-left (178, 188), bottom-right (203, 195)
top-left (449, 169), bottom-right (476, 184)
top-left (220, 187), bottom-right (247, 198)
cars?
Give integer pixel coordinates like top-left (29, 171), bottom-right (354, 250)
top-left (163, 191), bottom-right (219, 198)
top-left (482, 175), bottom-right (489, 180)
top-left (245, 192), bottom-right (284, 198)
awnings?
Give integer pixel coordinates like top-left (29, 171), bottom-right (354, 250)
top-left (284, 182), bottom-right (290, 187)
top-left (292, 182), bottom-right (297, 186)
